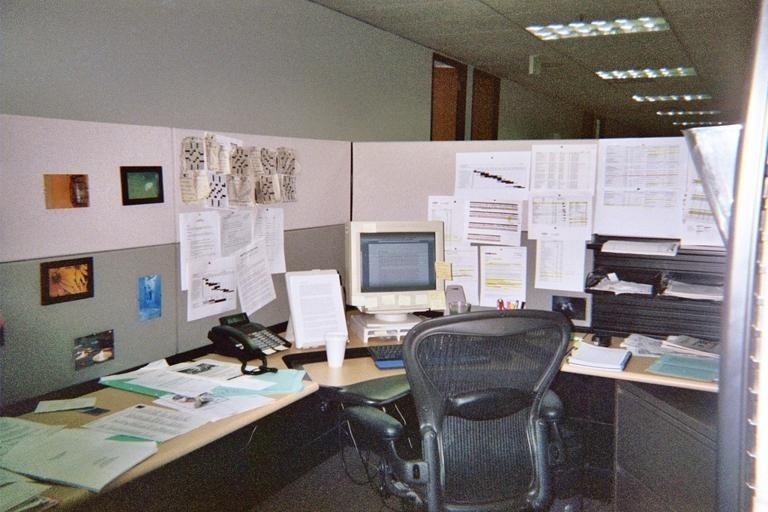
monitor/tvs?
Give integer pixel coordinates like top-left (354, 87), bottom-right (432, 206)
top-left (344, 220), bottom-right (445, 327)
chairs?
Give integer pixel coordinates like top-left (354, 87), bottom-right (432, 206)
top-left (337, 311), bottom-right (576, 510)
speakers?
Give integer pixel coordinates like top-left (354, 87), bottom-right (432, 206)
top-left (444, 285), bottom-right (468, 317)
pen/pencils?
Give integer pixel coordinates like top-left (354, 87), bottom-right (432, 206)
top-left (496, 299), bottom-right (526, 311)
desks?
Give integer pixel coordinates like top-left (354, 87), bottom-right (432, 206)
top-left (0, 310), bottom-right (721, 511)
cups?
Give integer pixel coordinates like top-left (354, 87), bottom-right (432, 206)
top-left (324, 329), bottom-right (347, 369)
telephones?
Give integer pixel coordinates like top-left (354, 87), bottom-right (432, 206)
top-left (207, 312), bottom-right (292, 361)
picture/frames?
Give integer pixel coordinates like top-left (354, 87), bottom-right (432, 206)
top-left (119, 165), bottom-right (164, 206)
top-left (39, 257), bottom-right (95, 306)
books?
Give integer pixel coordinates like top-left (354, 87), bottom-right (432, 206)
top-left (0, 357), bottom-right (277, 512)
top-left (661, 334), bottom-right (721, 360)
top-left (282, 268), bottom-right (350, 348)
top-left (567, 341), bottom-right (631, 372)
top-left (662, 278), bottom-right (726, 300)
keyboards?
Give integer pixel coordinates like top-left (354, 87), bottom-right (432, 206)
top-left (368, 345), bottom-right (411, 361)
